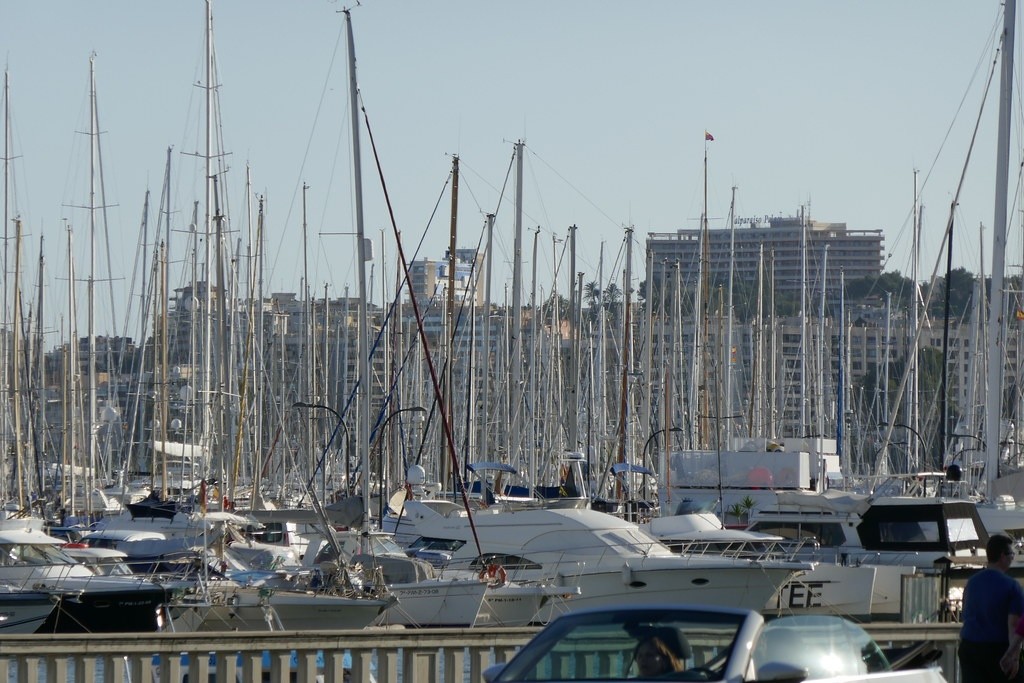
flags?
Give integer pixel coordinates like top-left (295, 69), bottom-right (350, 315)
top-left (706, 132), bottom-right (714, 141)
top-left (198, 480), bottom-right (206, 514)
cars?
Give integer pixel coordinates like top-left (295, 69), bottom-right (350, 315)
top-left (482, 600), bottom-right (894, 683)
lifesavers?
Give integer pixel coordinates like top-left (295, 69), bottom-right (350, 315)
top-left (479, 563), bottom-right (507, 590)
top-left (60, 543), bottom-right (90, 548)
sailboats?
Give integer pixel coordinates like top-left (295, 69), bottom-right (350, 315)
top-left (1, 0), bottom-right (1024, 637)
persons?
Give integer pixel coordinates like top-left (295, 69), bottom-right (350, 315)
top-left (957, 534), bottom-right (1024, 683)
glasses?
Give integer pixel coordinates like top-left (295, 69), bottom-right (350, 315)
top-left (1005, 551), bottom-right (1016, 555)
